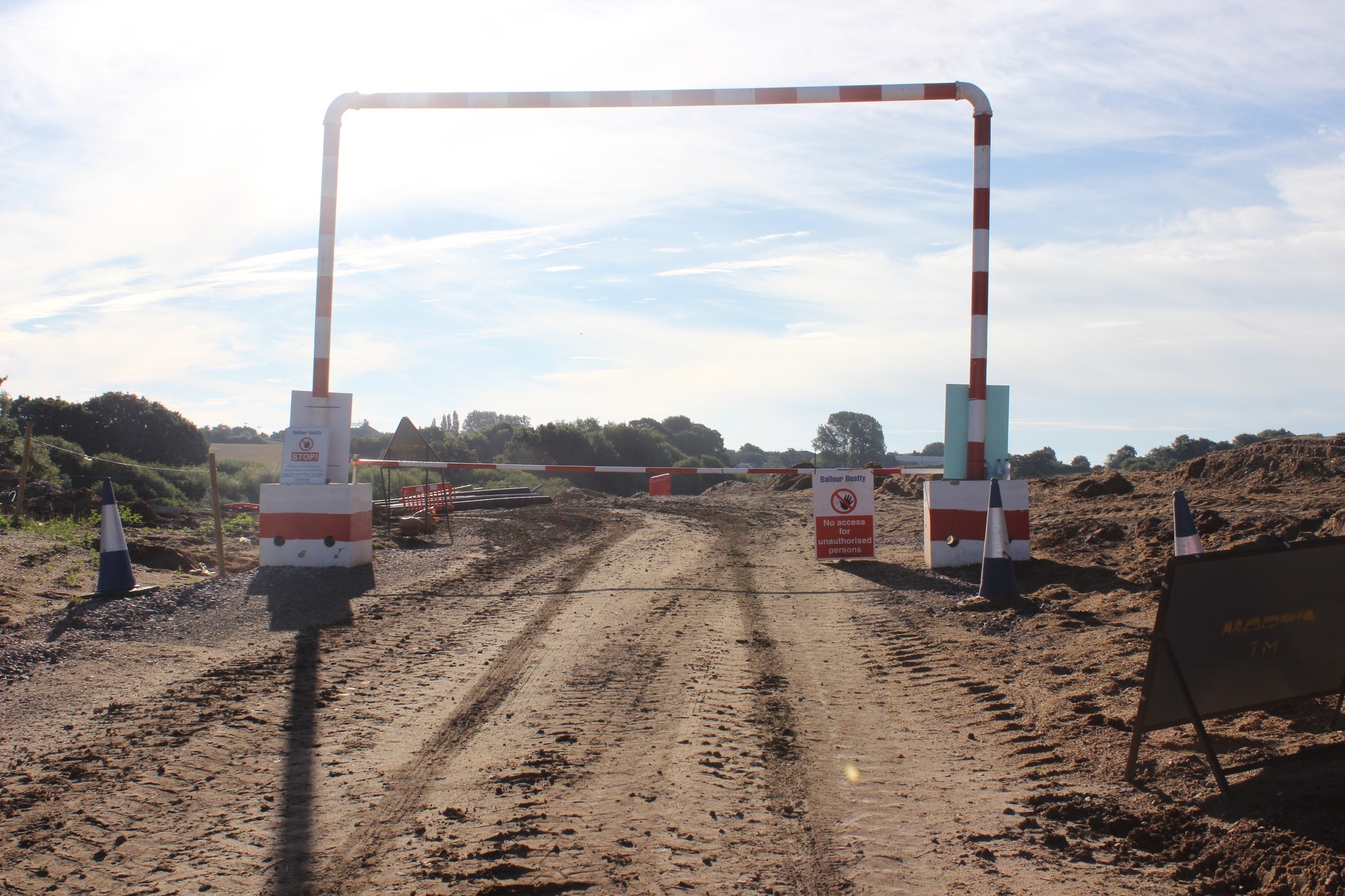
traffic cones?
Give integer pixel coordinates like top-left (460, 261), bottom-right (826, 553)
top-left (81, 476), bottom-right (160, 600)
top-left (1173, 489), bottom-right (1205, 556)
top-left (956, 478), bottom-right (1038, 611)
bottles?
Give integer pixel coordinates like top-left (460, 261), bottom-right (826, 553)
top-left (995, 459), bottom-right (1003, 480)
top-left (1003, 459), bottom-right (1010, 480)
top-left (984, 460), bottom-right (995, 480)
